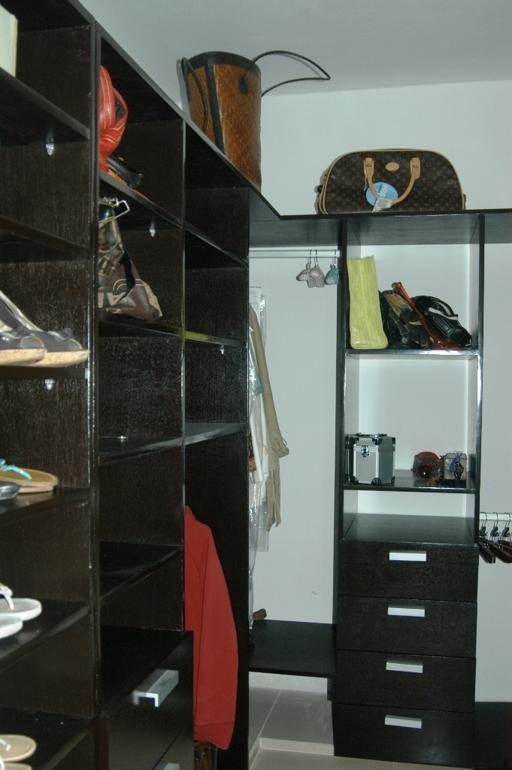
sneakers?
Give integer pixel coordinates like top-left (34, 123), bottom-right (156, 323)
top-left (0, 289), bottom-right (91, 371)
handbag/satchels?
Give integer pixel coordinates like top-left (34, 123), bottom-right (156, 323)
top-left (410, 296), bottom-right (473, 351)
top-left (96, 197), bottom-right (163, 321)
top-left (313, 149), bottom-right (466, 215)
top-left (346, 255), bottom-right (388, 351)
top-left (378, 289), bottom-right (437, 350)
top-left (181, 48), bottom-right (332, 192)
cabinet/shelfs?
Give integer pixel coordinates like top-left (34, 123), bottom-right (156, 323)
top-left (332, 205), bottom-right (510, 769)
top-left (0, 0), bottom-right (252, 770)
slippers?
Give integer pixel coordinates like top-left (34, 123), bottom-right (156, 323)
top-left (0, 458), bottom-right (59, 500)
top-left (0, 734), bottom-right (37, 770)
top-left (0, 583), bottom-right (42, 640)
